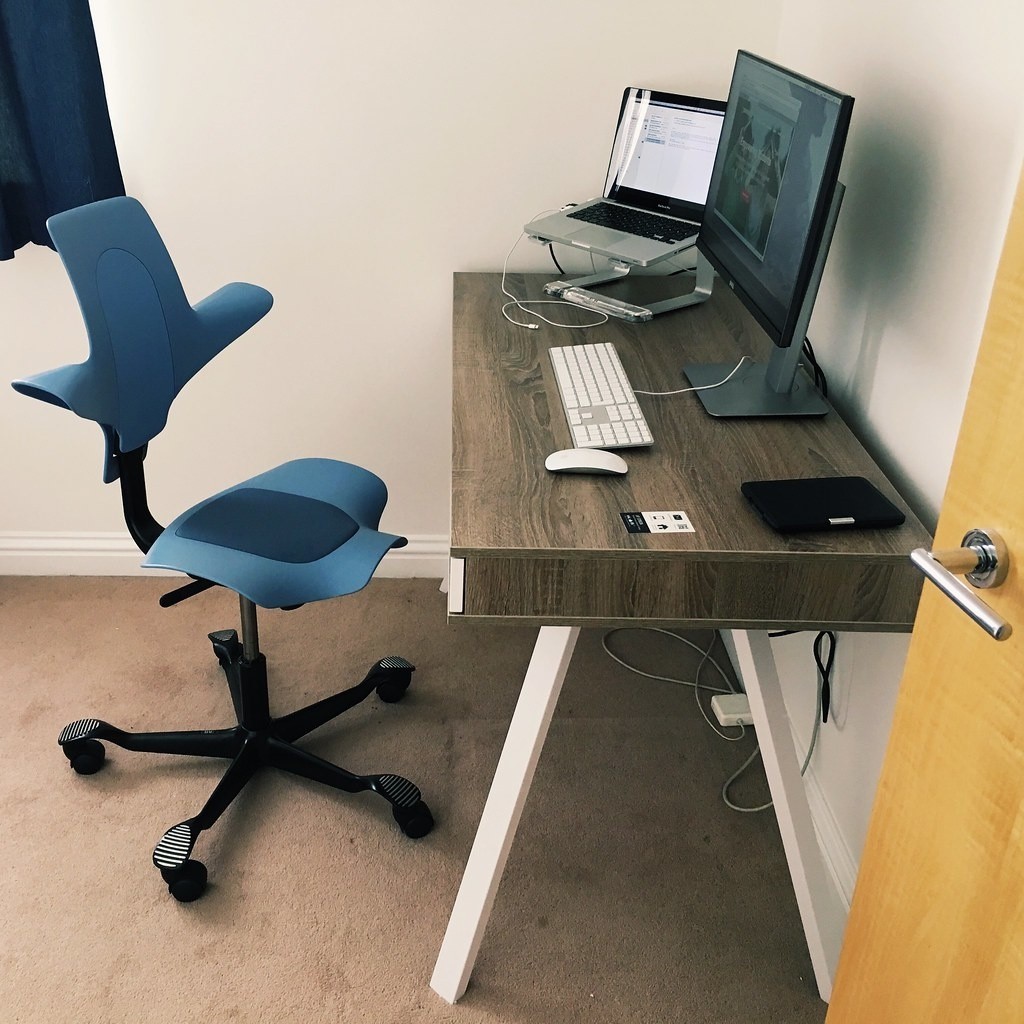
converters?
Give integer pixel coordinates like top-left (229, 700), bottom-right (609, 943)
top-left (710, 694), bottom-right (753, 726)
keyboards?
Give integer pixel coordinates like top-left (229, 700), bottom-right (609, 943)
top-left (547, 343), bottom-right (656, 449)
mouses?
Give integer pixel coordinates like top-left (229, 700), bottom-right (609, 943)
top-left (545, 448), bottom-right (628, 475)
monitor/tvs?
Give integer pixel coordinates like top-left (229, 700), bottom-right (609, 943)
top-left (683, 49), bottom-right (856, 421)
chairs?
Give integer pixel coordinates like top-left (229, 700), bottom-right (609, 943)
top-left (9, 197), bottom-right (443, 905)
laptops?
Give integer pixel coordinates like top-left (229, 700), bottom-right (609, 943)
top-left (523, 86), bottom-right (728, 266)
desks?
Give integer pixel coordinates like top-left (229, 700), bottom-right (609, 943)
top-left (429, 271), bottom-right (936, 1007)
